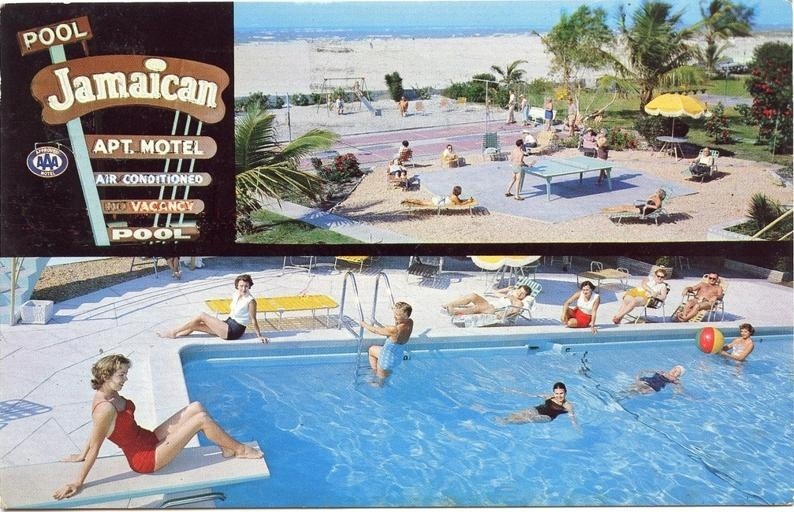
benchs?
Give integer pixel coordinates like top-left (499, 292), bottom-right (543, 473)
top-left (205, 294), bottom-right (340, 336)
top-left (528, 107), bottom-right (558, 125)
top-left (400, 197), bottom-right (480, 221)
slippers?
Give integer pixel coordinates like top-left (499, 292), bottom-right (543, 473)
top-left (505, 192), bottom-right (525, 201)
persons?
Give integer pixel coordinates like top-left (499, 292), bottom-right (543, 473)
top-left (631, 365), bottom-right (704, 407)
top-left (53, 354), bottom-right (264, 502)
top-left (156, 274), bottom-right (269, 344)
top-left (442, 284), bottom-right (532, 318)
top-left (162, 257), bottom-right (182, 279)
top-left (504, 89), bottom-right (610, 201)
top-left (395, 139), bottom-right (411, 160)
top-left (676, 273), bottom-right (723, 322)
top-left (399, 96), bottom-right (408, 117)
top-left (492, 383), bottom-right (582, 439)
top-left (688, 148), bottom-right (713, 174)
top-left (719, 323), bottom-right (755, 362)
top-left (360, 302), bottom-right (415, 388)
top-left (336, 91), bottom-right (348, 114)
top-left (612, 268), bottom-right (668, 324)
top-left (390, 159), bottom-right (406, 178)
top-left (326, 93), bottom-right (336, 112)
top-left (354, 81), bottom-right (363, 96)
top-left (600, 189), bottom-right (665, 215)
top-left (443, 144), bottom-right (457, 169)
top-left (401, 186), bottom-right (475, 207)
top-left (562, 280), bottom-right (601, 337)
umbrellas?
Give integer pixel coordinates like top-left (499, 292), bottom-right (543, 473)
top-left (646, 91), bottom-right (712, 157)
top-left (465, 253), bottom-right (540, 270)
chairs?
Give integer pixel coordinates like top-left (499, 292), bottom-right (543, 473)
top-left (621, 281), bottom-right (671, 326)
top-left (681, 149), bottom-right (720, 183)
top-left (607, 185), bottom-right (672, 227)
top-left (391, 148), bottom-right (414, 167)
top-left (670, 274), bottom-right (729, 324)
top-left (386, 161), bottom-right (408, 188)
top-left (333, 256), bottom-right (372, 275)
top-left (280, 256), bottom-right (316, 277)
top-left (525, 131), bottom-right (553, 155)
top-left (450, 276), bottom-right (542, 328)
top-left (404, 256), bottom-right (442, 288)
top-left (482, 133), bottom-right (501, 162)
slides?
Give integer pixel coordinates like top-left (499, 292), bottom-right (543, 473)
top-left (354, 90), bottom-right (381, 117)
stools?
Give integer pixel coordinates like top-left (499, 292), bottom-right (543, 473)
top-left (577, 261), bottom-right (631, 294)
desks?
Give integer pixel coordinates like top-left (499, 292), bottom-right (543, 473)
top-left (655, 136), bottom-right (688, 163)
top-left (519, 155), bottom-right (617, 201)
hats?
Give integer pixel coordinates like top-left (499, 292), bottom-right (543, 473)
top-left (674, 365), bottom-right (686, 377)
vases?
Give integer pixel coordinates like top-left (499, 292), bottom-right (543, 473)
top-left (20, 300), bottom-right (54, 324)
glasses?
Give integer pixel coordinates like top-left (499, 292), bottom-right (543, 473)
top-left (656, 275), bottom-right (664, 278)
top-left (709, 277), bottom-right (717, 281)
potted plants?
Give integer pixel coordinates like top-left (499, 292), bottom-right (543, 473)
top-left (767, 250), bottom-right (789, 284)
top-left (650, 255), bottom-right (674, 279)
